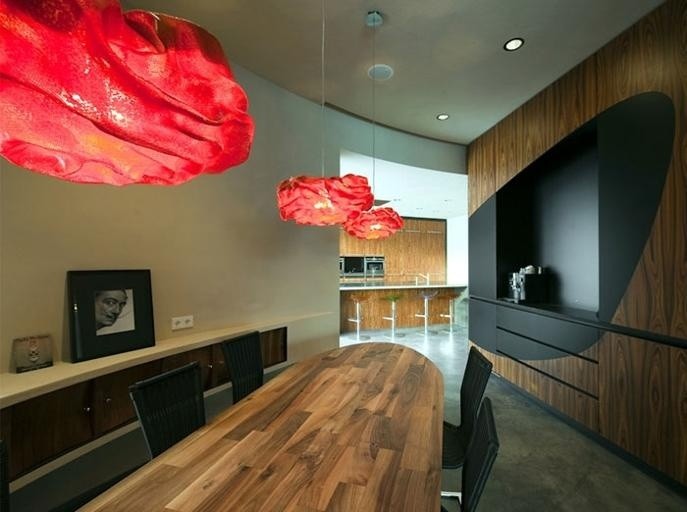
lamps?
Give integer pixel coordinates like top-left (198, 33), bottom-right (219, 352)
top-left (338, 6), bottom-right (406, 242)
top-left (0, 1), bottom-right (257, 194)
top-left (273, 0), bottom-right (374, 228)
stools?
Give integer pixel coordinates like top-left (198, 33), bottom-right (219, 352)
top-left (344, 289), bottom-right (461, 341)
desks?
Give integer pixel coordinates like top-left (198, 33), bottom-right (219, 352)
top-left (62, 339), bottom-right (450, 512)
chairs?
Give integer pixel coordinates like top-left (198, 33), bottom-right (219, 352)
top-left (440, 346), bottom-right (494, 510)
top-left (220, 329), bottom-right (264, 407)
top-left (124, 358), bottom-right (206, 463)
top-left (434, 397), bottom-right (501, 510)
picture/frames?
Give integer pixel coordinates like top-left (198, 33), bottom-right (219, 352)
top-left (60, 267), bottom-right (157, 364)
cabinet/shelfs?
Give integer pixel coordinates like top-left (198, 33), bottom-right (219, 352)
top-left (2, 314), bottom-right (297, 495)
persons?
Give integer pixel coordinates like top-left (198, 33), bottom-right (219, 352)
top-left (93, 290), bottom-right (130, 332)
